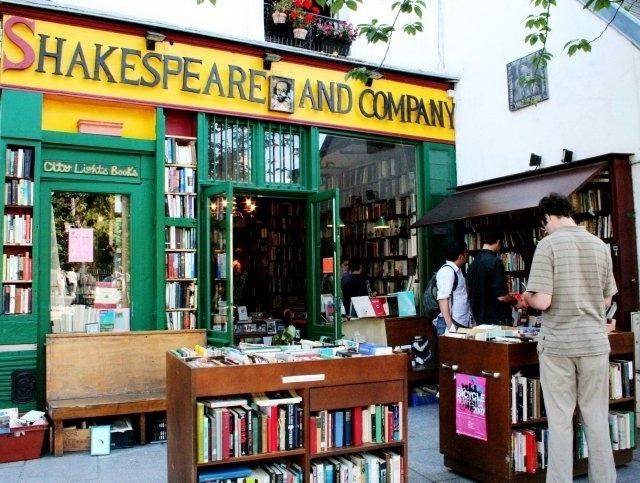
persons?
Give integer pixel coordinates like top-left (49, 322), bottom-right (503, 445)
top-left (518, 191), bottom-right (620, 483)
top-left (435, 240), bottom-right (470, 337)
top-left (271, 80), bottom-right (292, 111)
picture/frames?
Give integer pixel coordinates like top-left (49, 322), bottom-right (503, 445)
top-left (267, 74), bottom-right (296, 114)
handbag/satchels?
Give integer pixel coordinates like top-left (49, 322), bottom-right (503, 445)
top-left (422, 265), bottom-right (459, 320)
top-left (411, 385), bottom-right (439, 406)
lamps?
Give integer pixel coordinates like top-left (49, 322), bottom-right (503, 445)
top-left (208, 192), bottom-right (259, 221)
top-left (260, 50), bottom-right (285, 70)
top-left (364, 68), bottom-right (383, 85)
top-left (529, 150), bottom-right (545, 170)
top-left (143, 29), bottom-right (167, 51)
top-left (559, 148), bottom-right (574, 163)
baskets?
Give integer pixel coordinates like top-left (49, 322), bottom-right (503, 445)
top-left (151, 418), bottom-right (167, 441)
top-left (92, 416), bottom-right (135, 449)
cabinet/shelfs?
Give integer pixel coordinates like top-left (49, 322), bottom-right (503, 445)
top-left (163, 133), bottom-right (201, 328)
top-left (433, 323), bottom-right (638, 483)
top-left (408, 151), bottom-right (637, 328)
top-left (234, 149), bottom-right (417, 344)
top-left (0, 142), bottom-right (40, 321)
top-left (79, 257), bottom-right (111, 307)
top-left (167, 346), bottom-right (411, 483)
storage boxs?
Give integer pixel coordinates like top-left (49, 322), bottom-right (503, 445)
top-left (0, 409), bottom-right (49, 464)
top-left (48, 420), bottom-right (97, 455)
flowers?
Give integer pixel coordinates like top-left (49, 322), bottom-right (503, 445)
top-left (270, 0), bottom-right (356, 48)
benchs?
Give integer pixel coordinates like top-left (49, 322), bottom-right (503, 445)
top-left (42, 326), bottom-right (211, 457)
